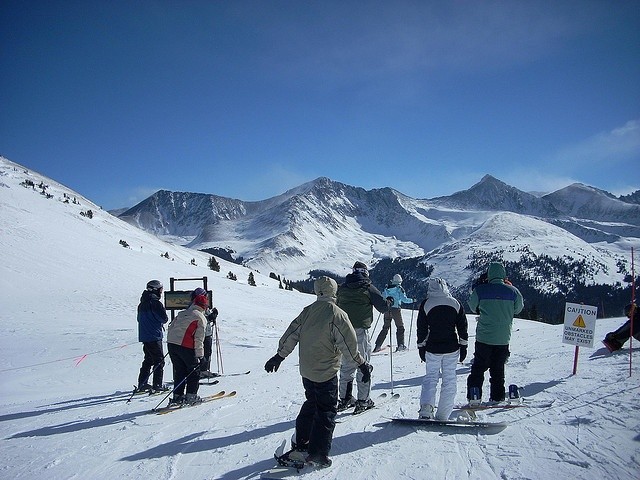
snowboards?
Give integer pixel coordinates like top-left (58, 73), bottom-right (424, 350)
top-left (260, 450), bottom-right (311, 480)
top-left (435, 401), bottom-right (553, 408)
top-left (390, 416), bottom-right (507, 428)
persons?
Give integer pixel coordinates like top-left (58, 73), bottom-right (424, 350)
top-left (137, 280), bottom-right (168, 391)
top-left (605, 303), bottom-right (640, 351)
top-left (168, 295), bottom-right (209, 406)
top-left (264, 276), bottom-right (373, 467)
top-left (373, 274), bottom-right (417, 353)
top-left (466, 262), bottom-right (524, 405)
top-left (190, 288), bottom-right (219, 376)
top-left (337, 261), bottom-right (394, 409)
top-left (417, 277), bottom-right (468, 420)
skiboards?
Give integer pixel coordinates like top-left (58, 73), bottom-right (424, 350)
top-left (156, 391), bottom-right (236, 415)
top-left (370, 344), bottom-right (409, 356)
top-left (165, 371), bottom-right (251, 386)
top-left (335, 392), bottom-right (399, 424)
top-left (129, 387), bottom-right (173, 396)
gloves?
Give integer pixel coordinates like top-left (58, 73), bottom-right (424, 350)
top-left (208, 313), bottom-right (216, 320)
top-left (212, 308), bottom-right (218, 317)
top-left (359, 361), bottom-right (373, 383)
top-left (412, 299), bottom-right (417, 303)
top-left (200, 357), bottom-right (207, 367)
top-left (386, 296), bottom-right (394, 306)
top-left (459, 342), bottom-right (468, 362)
top-left (419, 344), bottom-right (427, 362)
top-left (265, 353), bottom-right (285, 373)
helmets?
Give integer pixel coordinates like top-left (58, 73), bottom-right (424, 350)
top-left (624, 303), bottom-right (636, 317)
top-left (147, 280), bottom-right (163, 296)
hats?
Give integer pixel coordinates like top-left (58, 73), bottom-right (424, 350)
top-left (193, 288), bottom-right (207, 298)
top-left (392, 274), bottom-right (403, 284)
top-left (353, 261), bottom-right (367, 275)
top-left (195, 295), bottom-right (208, 310)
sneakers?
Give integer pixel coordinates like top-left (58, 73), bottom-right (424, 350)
top-left (173, 394), bottom-right (186, 403)
top-left (295, 443), bottom-right (308, 452)
top-left (306, 454), bottom-right (332, 467)
top-left (153, 384), bottom-right (164, 391)
top-left (341, 396), bottom-right (357, 408)
top-left (138, 383), bottom-right (152, 390)
top-left (490, 396), bottom-right (504, 403)
top-left (374, 346), bottom-right (382, 353)
top-left (435, 410), bottom-right (448, 421)
top-left (185, 394), bottom-right (203, 404)
top-left (605, 333), bottom-right (623, 350)
top-left (355, 399), bottom-right (375, 411)
top-left (398, 344), bottom-right (406, 350)
top-left (467, 389), bottom-right (482, 405)
top-left (419, 405), bottom-right (434, 419)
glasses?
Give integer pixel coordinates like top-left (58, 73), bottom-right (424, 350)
top-left (159, 288), bottom-right (163, 293)
top-left (354, 267), bottom-right (369, 277)
